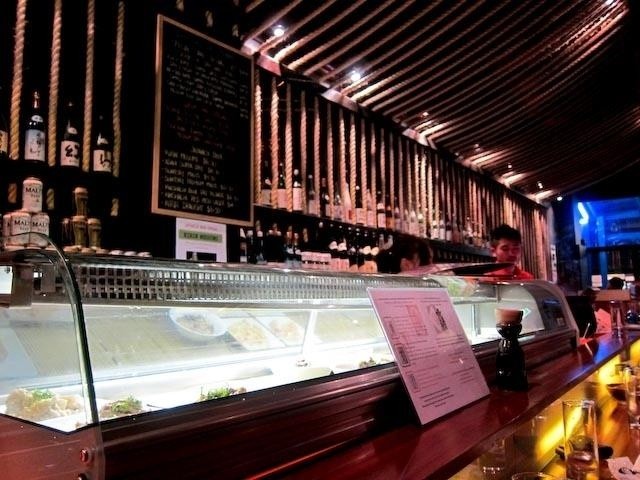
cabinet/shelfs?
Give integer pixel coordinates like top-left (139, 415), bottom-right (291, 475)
top-left (0, 232), bottom-right (580, 480)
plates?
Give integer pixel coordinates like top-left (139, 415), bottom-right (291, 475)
top-left (254, 314), bottom-right (322, 345)
top-left (222, 316), bottom-right (285, 353)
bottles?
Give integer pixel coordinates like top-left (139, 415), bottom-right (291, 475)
top-left (59, 101), bottom-right (82, 172)
top-left (25, 89), bottom-right (47, 165)
top-left (494, 307), bottom-right (530, 394)
top-left (91, 115), bottom-right (113, 174)
top-left (238, 157), bottom-right (494, 274)
top-left (1, 84), bottom-right (10, 159)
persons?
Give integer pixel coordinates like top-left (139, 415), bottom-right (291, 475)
top-left (380, 233), bottom-right (441, 277)
top-left (607, 277), bottom-right (624, 290)
top-left (478, 223), bottom-right (536, 283)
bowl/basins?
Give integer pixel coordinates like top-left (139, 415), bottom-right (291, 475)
top-left (168, 307), bottom-right (228, 344)
top-left (606, 382), bottom-right (640, 403)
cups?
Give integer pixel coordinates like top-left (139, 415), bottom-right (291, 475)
top-left (563, 399), bottom-right (601, 480)
top-left (624, 366), bottom-right (640, 429)
top-left (477, 438), bottom-right (507, 474)
top-left (512, 471), bottom-right (558, 480)
top-left (610, 299), bottom-right (628, 339)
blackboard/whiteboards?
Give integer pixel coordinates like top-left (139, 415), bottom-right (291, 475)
top-left (151, 14), bottom-right (255, 229)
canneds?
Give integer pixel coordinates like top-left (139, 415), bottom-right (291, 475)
top-left (2, 175), bottom-right (152, 258)
